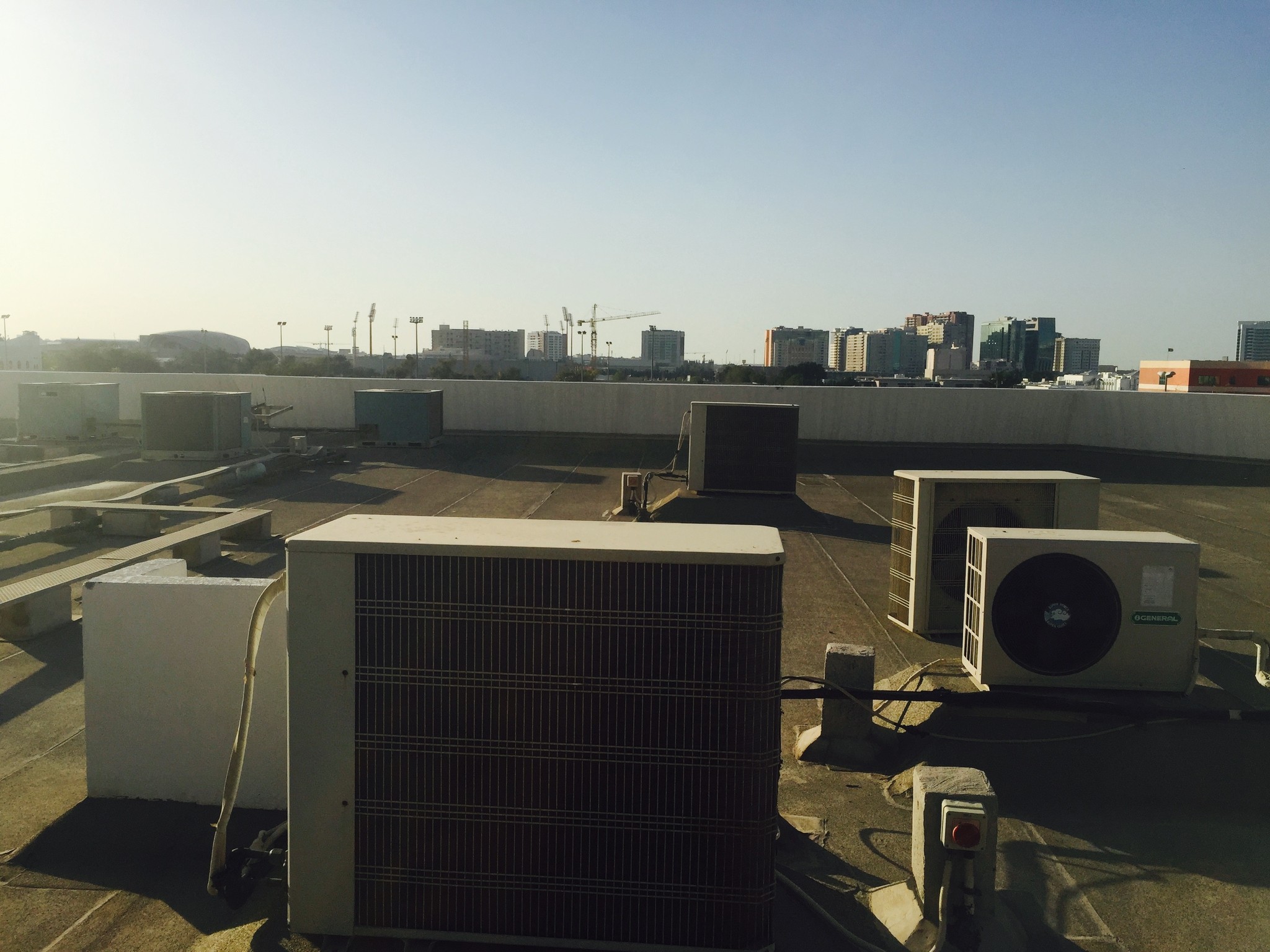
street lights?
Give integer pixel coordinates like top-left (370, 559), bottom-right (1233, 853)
top-left (1, 314), bottom-right (11, 371)
top-left (606, 341), bottom-right (612, 381)
top-left (324, 325), bottom-right (333, 358)
top-left (649, 325), bottom-right (657, 381)
top-left (277, 322), bottom-right (286, 363)
top-left (577, 331), bottom-right (586, 382)
top-left (201, 330), bottom-right (208, 374)
top-left (410, 317), bottom-right (423, 366)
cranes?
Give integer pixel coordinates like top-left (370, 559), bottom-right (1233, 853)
top-left (684, 352), bottom-right (710, 364)
top-left (577, 304), bottom-right (662, 369)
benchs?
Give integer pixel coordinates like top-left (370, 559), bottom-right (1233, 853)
top-left (0, 449), bottom-right (289, 637)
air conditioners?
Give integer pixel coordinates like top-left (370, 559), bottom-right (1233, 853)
top-left (883, 462), bottom-right (1103, 636)
top-left (615, 395), bottom-right (803, 516)
top-left (139, 387), bottom-right (255, 460)
top-left (958, 520), bottom-right (1207, 702)
top-left (206, 508), bottom-right (812, 952)
